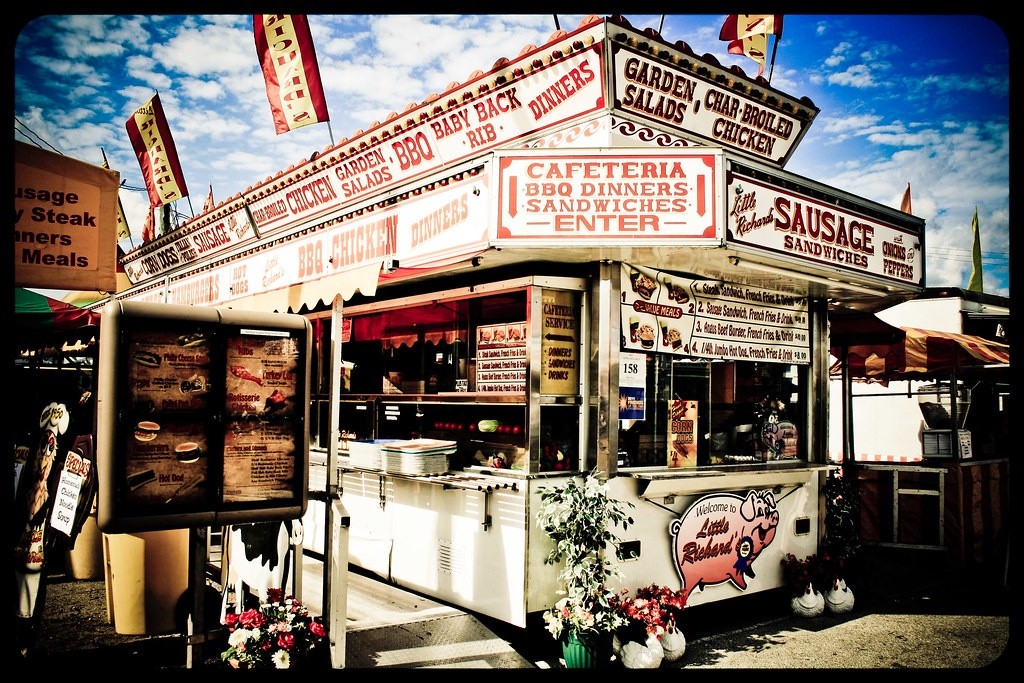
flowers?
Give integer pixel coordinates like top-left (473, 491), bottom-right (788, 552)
top-left (780, 551), bottom-right (846, 597)
top-left (542, 468), bottom-right (687, 642)
top-left (221, 588), bottom-right (325, 669)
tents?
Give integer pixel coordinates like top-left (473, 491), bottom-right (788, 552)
top-left (14, 287), bottom-right (100, 372)
top-left (829, 322), bottom-right (1011, 464)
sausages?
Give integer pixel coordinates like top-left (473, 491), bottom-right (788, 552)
top-left (175, 474), bottom-right (204, 496)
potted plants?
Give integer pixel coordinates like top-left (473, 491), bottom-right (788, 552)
top-left (819, 455), bottom-right (871, 613)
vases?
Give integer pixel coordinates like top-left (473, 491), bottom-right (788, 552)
top-left (791, 584), bottom-right (823, 617)
top-left (562, 629), bottom-right (686, 669)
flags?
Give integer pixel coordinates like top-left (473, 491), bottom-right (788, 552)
top-left (141, 204), bottom-right (152, 241)
top-left (900, 183), bottom-right (911, 215)
top-left (967, 212), bottom-right (983, 293)
top-left (202, 186), bottom-right (214, 212)
top-left (126, 94), bottom-right (188, 206)
top-left (718, 15), bottom-right (783, 42)
top-left (100, 162), bottom-right (130, 239)
top-left (251, 13), bottom-right (329, 136)
top-left (727, 32), bottom-right (767, 67)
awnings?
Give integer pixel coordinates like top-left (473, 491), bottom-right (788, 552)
top-left (343, 301), bottom-right (469, 347)
top-left (208, 249), bottom-right (491, 314)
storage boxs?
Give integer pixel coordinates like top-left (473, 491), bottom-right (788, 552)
top-left (347, 438), bottom-right (397, 471)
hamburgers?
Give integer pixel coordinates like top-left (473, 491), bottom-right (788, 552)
top-left (175, 443), bottom-right (202, 463)
top-left (133, 421), bottom-right (160, 442)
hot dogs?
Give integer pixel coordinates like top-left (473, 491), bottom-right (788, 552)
top-left (127, 469), bottom-right (157, 490)
top-left (177, 333), bottom-right (206, 349)
top-left (131, 350), bottom-right (161, 368)
